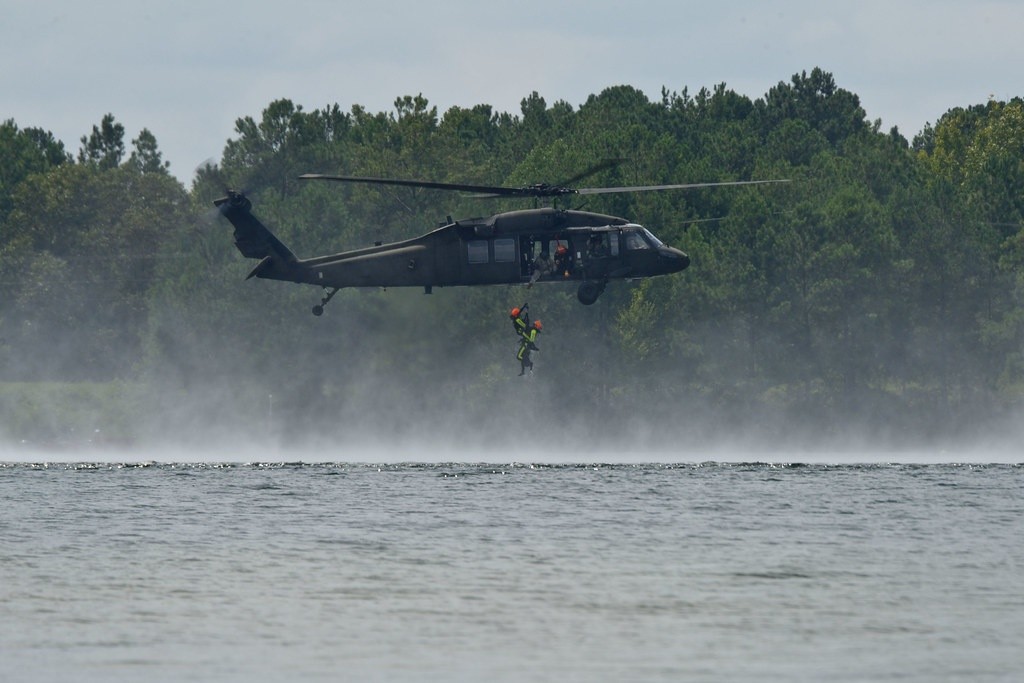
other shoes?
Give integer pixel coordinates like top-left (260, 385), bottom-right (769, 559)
top-left (527, 283), bottom-right (533, 289)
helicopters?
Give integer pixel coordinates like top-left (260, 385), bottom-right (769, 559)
top-left (209, 156), bottom-right (794, 315)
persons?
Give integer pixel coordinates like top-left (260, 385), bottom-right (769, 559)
top-left (510, 307), bottom-right (540, 351)
top-left (528, 250), bottom-right (558, 290)
top-left (553, 245), bottom-right (575, 278)
top-left (626, 232), bottom-right (641, 250)
top-left (517, 321), bottom-right (543, 377)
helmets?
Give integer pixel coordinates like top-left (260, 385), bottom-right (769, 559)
top-left (534, 321), bottom-right (541, 328)
top-left (512, 308), bottom-right (519, 315)
top-left (557, 245), bottom-right (566, 254)
